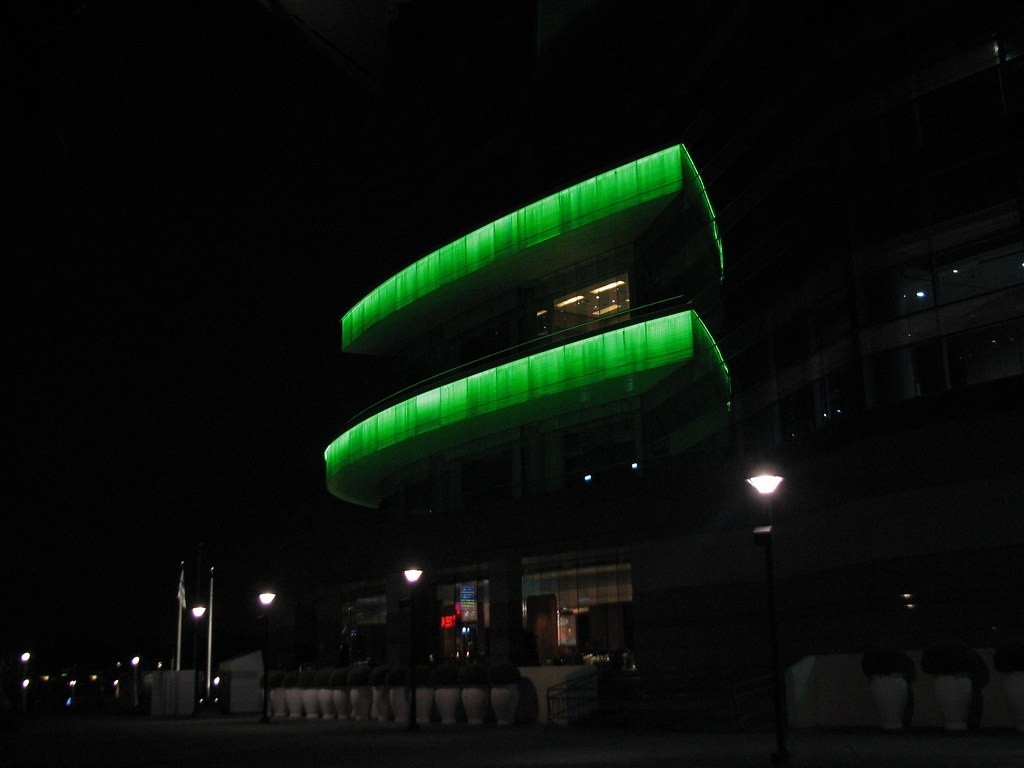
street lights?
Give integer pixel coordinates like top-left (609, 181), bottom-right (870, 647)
top-left (259, 592), bottom-right (277, 723)
top-left (189, 601), bottom-right (206, 719)
top-left (401, 564), bottom-right (425, 730)
top-left (741, 467), bottom-right (796, 766)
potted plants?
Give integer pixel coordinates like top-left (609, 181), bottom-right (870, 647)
top-left (862, 646), bottom-right (915, 728)
top-left (993, 637), bottom-right (1024, 732)
top-left (432, 665), bottom-right (462, 724)
top-left (297, 670), bottom-right (321, 719)
top-left (284, 671), bottom-right (302, 718)
top-left (347, 666), bottom-right (371, 721)
top-left (485, 660), bottom-right (521, 727)
top-left (313, 668), bottom-right (337, 720)
top-left (921, 642), bottom-right (985, 729)
top-left (267, 671), bottom-right (287, 718)
top-left (458, 669), bottom-right (486, 724)
top-left (414, 665), bottom-right (436, 723)
top-left (370, 665), bottom-right (391, 721)
top-left (330, 668), bottom-right (351, 721)
top-left (389, 665), bottom-right (413, 721)
